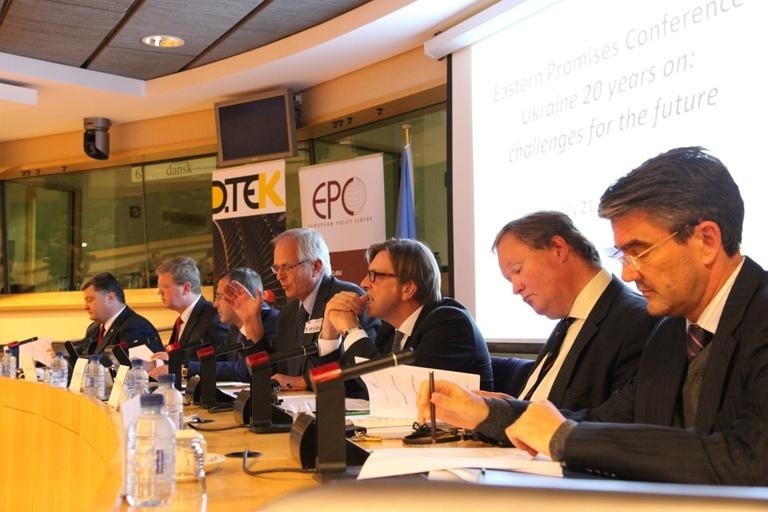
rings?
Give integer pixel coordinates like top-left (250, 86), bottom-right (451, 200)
top-left (287, 384), bottom-right (294, 389)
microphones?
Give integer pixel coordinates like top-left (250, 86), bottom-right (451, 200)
top-left (341, 346), bottom-right (416, 380)
top-left (268, 342), bottom-right (319, 365)
top-left (130, 336), bottom-right (159, 348)
top-left (213, 339), bottom-right (253, 357)
top-left (19, 337), bottom-right (38, 345)
top-left (74, 336), bottom-right (97, 349)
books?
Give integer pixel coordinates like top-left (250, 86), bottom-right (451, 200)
top-left (421, 470), bottom-right (468, 483)
top-left (351, 415), bottom-right (449, 435)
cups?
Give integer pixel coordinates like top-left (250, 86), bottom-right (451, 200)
top-left (174, 426), bottom-right (209, 502)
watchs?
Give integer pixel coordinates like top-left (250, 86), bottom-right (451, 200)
top-left (182, 364), bottom-right (188, 378)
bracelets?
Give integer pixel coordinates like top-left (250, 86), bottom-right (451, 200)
top-left (343, 326), bottom-right (362, 337)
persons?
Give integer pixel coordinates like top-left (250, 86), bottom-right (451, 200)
top-left (46, 272), bottom-right (164, 390)
top-left (151, 255), bottom-right (231, 360)
top-left (223, 228), bottom-right (380, 391)
top-left (415, 146), bottom-right (767, 487)
top-left (317, 237), bottom-right (493, 402)
top-left (468, 210), bottom-right (666, 412)
top-left (148, 267), bottom-right (280, 383)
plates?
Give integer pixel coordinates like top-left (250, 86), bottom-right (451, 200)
top-left (203, 451), bottom-right (225, 472)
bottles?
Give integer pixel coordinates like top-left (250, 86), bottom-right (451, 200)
top-left (126, 358), bottom-right (150, 394)
top-left (49, 352), bottom-right (69, 389)
top-left (0, 349), bottom-right (17, 378)
top-left (123, 393), bottom-right (177, 507)
top-left (152, 372), bottom-right (184, 431)
top-left (83, 355), bottom-right (106, 401)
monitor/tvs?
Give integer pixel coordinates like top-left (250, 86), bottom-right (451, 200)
top-left (213, 87), bottom-right (298, 168)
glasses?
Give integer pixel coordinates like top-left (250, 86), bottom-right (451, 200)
top-left (608, 221), bottom-right (701, 270)
top-left (369, 270), bottom-right (397, 282)
top-left (270, 259), bottom-right (308, 274)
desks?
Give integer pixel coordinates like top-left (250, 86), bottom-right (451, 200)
top-left (0, 341), bottom-right (498, 510)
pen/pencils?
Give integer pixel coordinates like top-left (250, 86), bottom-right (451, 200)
top-left (430, 371), bottom-right (436, 446)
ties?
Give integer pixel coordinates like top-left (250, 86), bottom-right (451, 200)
top-left (175, 317), bottom-right (184, 342)
top-left (390, 331), bottom-right (405, 354)
top-left (523, 317), bottom-right (577, 401)
top-left (297, 304), bottom-right (309, 336)
top-left (686, 325), bottom-right (711, 362)
top-left (98, 324), bottom-right (105, 348)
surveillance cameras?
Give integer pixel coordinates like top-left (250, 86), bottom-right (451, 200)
top-left (83, 118), bottom-right (111, 160)
